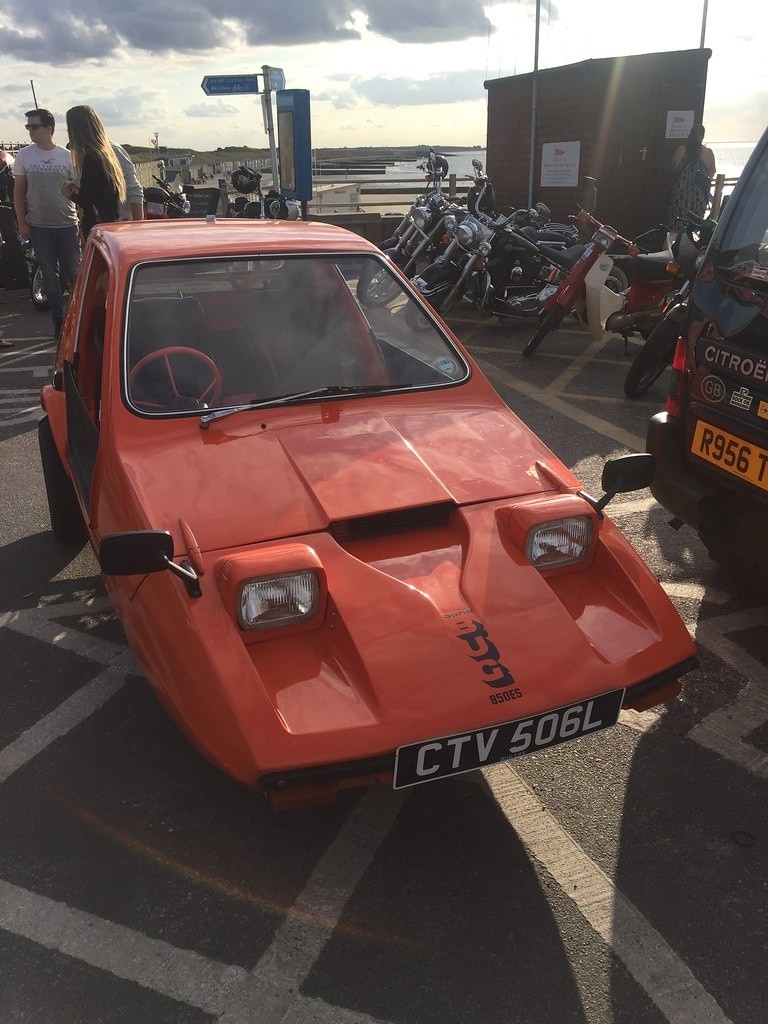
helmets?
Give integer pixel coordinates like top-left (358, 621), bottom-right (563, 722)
top-left (467, 184), bottom-right (495, 214)
top-left (427, 155), bottom-right (448, 180)
top-left (232, 167), bottom-right (261, 194)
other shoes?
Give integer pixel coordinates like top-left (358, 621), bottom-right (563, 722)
top-left (0, 339), bottom-right (14, 347)
top-left (54, 319), bottom-right (64, 338)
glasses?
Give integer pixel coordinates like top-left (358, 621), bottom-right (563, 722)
top-left (25, 125), bottom-right (46, 130)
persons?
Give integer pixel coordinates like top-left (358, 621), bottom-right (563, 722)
top-left (108, 140), bottom-right (143, 220)
top-left (663, 124), bottom-right (715, 250)
top-left (0, 150), bottom-right (31, 290)
top-left (62, 106), bottom-right (126, 244)
top-left (13, 109), bottom-right (80, 338)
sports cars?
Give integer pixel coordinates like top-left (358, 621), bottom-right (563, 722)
top-left (34, 215), bottom-right (700, 814)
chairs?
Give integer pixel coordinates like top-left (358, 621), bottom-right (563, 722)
top-left (251, 290), bottom-right (355, 383)
top-left (124, 295), bottom-right (214, 393)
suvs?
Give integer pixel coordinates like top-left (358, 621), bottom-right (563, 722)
top-left (640, 124), bottom-right (768, 580)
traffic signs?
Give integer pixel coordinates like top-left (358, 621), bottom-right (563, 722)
top-left (200, 74), bottom-right (258, 96)
top-left (268, 67), bottom-right (286, 91)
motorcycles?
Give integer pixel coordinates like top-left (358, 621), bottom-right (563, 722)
top-left (355, 144), bottom-right (718, 398)
top-left (22, 163), bottom-right (300, 309)
